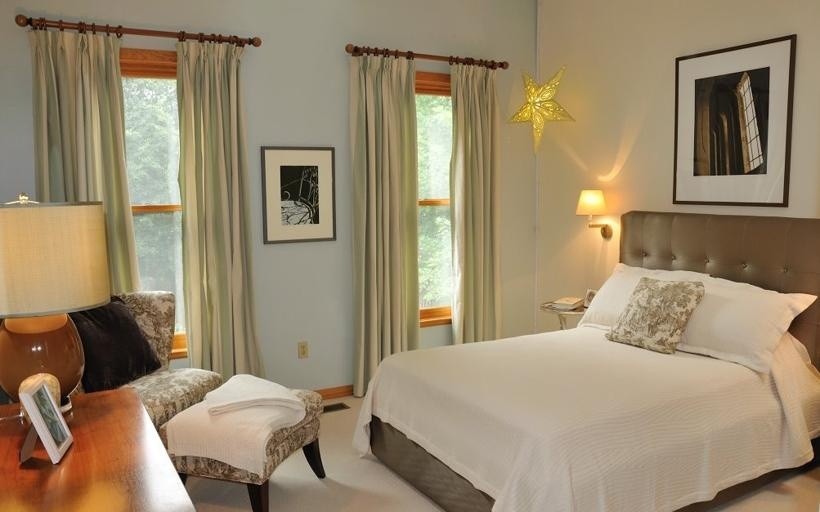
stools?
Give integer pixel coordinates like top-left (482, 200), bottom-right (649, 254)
top-left (159, 385), bottom-right (328, 512)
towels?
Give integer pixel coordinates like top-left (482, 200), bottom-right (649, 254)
top-left (163, 369), bottom-right (309, 482)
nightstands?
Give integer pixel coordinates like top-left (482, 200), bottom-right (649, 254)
top-left (533, 298), bottom-right (590, 328)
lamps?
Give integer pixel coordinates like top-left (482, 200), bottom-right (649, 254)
top-left (506, 65), bottom-right (576, 154)
top-left (576, 187), bottom-right (613, 241)
top-left (0, 189), bottom-right (116, 419)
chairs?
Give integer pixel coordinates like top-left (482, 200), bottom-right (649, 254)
top-left (64, 288), bottom-right (228, 433)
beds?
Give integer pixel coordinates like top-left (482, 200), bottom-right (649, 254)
top-left (347, 208), bottom-right (818, 511)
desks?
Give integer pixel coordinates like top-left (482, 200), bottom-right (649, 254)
top-left (0, 386), bottom-right (200, 512)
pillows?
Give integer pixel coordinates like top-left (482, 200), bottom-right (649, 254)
top-left (66, 294), bottom-right (166, 391)
top-left (675, 273), bottom-right (819, 375)
top-left (572, 259), bottom-right (711, 332)
top-left (603, 274), bottom-right (707, 354)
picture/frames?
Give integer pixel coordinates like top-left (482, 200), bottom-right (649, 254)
top-left (260, 145), bottom-right (337, 244)
top-left (673, 34), bottom-right (798, 208)
top-left (17, 377), bottom-right (75, 464)
